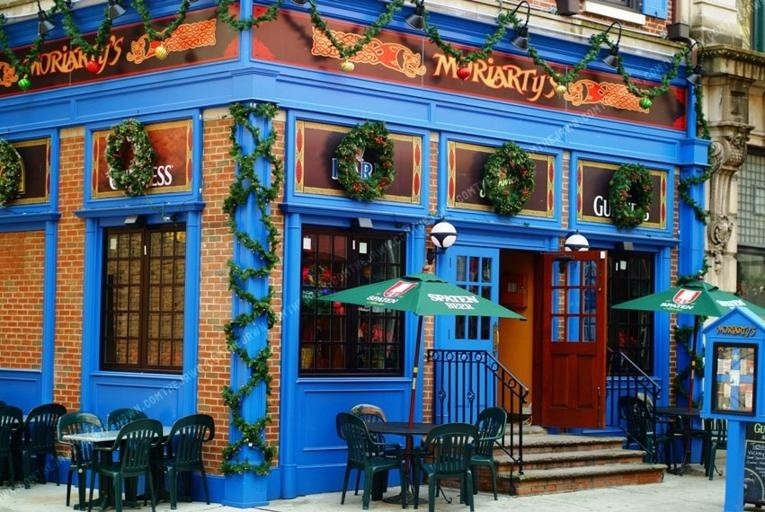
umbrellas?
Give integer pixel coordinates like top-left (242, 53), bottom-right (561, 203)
top-left (313, 264), bottom-right (528, 507)
top-left (605, 279), bottom-right (765, 470)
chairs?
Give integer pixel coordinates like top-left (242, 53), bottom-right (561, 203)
top-left (618, 395), bottom-right (727, 480)
top-left (0, 399), bottom-right (67, 491)
top-left (105, 408), bottom-right (167, 503)
top-left (353, 404), bottom-right (410, 503)
top-left (87, 420), bottom-right (163, 512)
top-left (414, 424), bottom-right (478, 512)
top-left (337, 413), bottom-right (409, 510)
top-left (56, 412), bottom-right (120, 508)
top-left (435, 407), bottom-right (507, 504)
top-left (144, 414), bottom-right (215, 510)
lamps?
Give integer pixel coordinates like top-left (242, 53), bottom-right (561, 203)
top-left (506, 1), bottom-right (532, 51)
top-left (679, 40), bottom-right (705, 88)
top-left (592, 20), bottom-right (623, 69)
top-left (31, 0), bottom-right (128, 35)
top-left (404, 1), bottom-right (428, 31)
top-left (559, 227), bottom-right (590, 272)
top-left (426, 216), bottom-right (459, 264)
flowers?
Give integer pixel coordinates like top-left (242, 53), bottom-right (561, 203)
top-left (337, 120), bottom-right (397, 203)
top-left (101, 119), bottom-right (155, 198)
top-left (608, 163), bottom-right (655, 229)
top-left (0, 137), bottom-right (21, 208)
top-left (484, 141), bottom-right (536, 218)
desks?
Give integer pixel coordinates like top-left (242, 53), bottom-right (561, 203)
top-left (61, 426), bottom-right (203, 512)
top-left (364, 420), bottom-right (454, 506)
